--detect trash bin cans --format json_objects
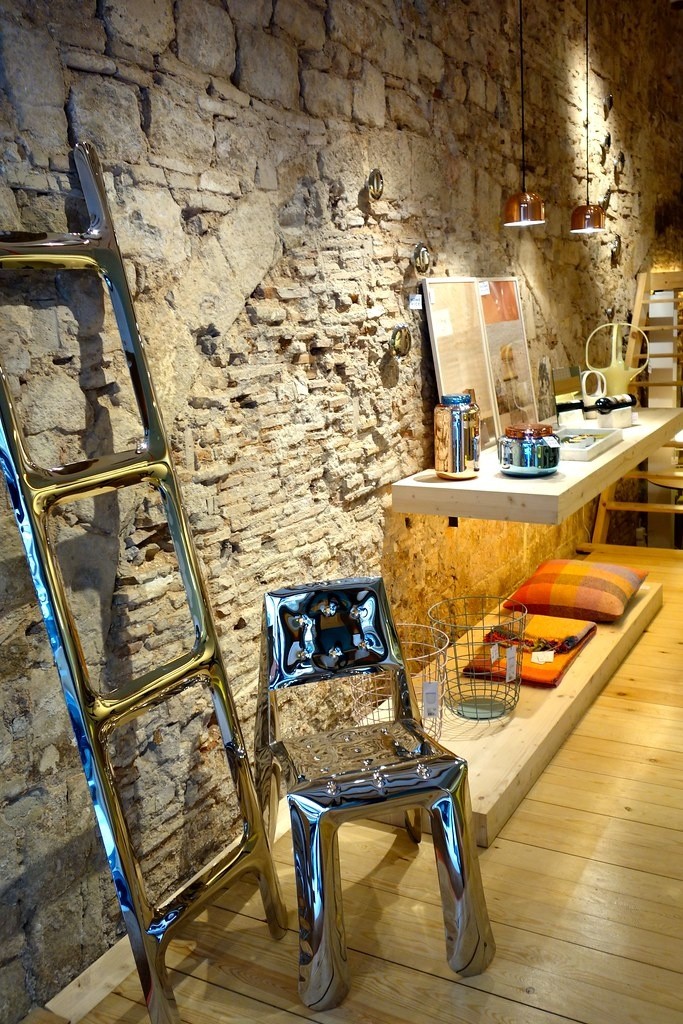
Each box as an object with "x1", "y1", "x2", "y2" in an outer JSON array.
[
  {"x1": 352, "y1": 625, "x2": 450, "y2": 745},
  {"x1": 426, "y1": 596, "x2": 528, "y2": 721}
]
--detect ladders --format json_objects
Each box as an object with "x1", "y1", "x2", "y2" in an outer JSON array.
[
  {"x1": 592, "y1": 272, "x2": 683, "y2": 558},
  {"x1": 0, "y1": 138, "x2": 292, "y2": 1024}
]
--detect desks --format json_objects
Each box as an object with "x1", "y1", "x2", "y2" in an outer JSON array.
[{"x1": 388, "y1": 405, "x2": 683, "y2": 524}]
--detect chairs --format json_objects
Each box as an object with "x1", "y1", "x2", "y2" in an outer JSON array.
[{"x1": 242, "y1": 576, "x2": 494, "y2": 1011}]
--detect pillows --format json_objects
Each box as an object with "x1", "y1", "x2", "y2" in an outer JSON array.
[{"x1": 506, "y1": 559, "x2": 650, "y2": 623}]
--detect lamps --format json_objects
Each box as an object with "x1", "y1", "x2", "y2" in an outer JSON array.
[
  {"x1": 503, "y1": 0, "x2": 545, "y2": 226},
  {"x1": 572, "y1": 0, "x2": 607, "y2": 233}
]
